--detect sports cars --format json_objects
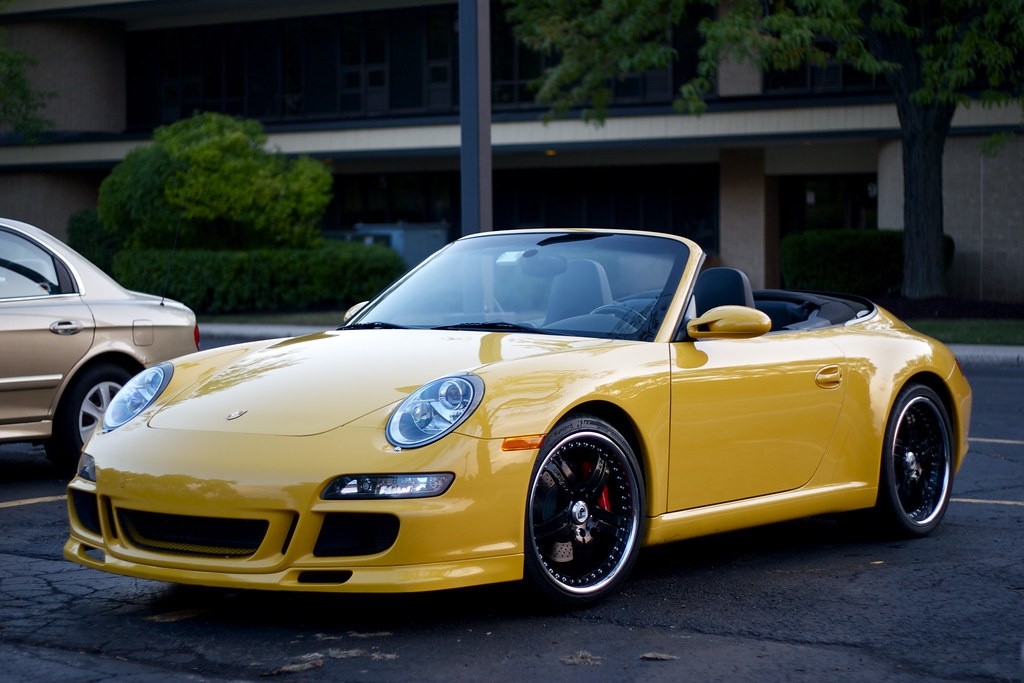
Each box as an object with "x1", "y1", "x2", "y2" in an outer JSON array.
[{"x1": 64, "y1": 225, "x2": 974, "y2": 613}]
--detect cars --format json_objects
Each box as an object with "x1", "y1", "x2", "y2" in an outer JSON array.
[{"x1": 1, "y1": 218, "x2": 201, "y2": 486}]
